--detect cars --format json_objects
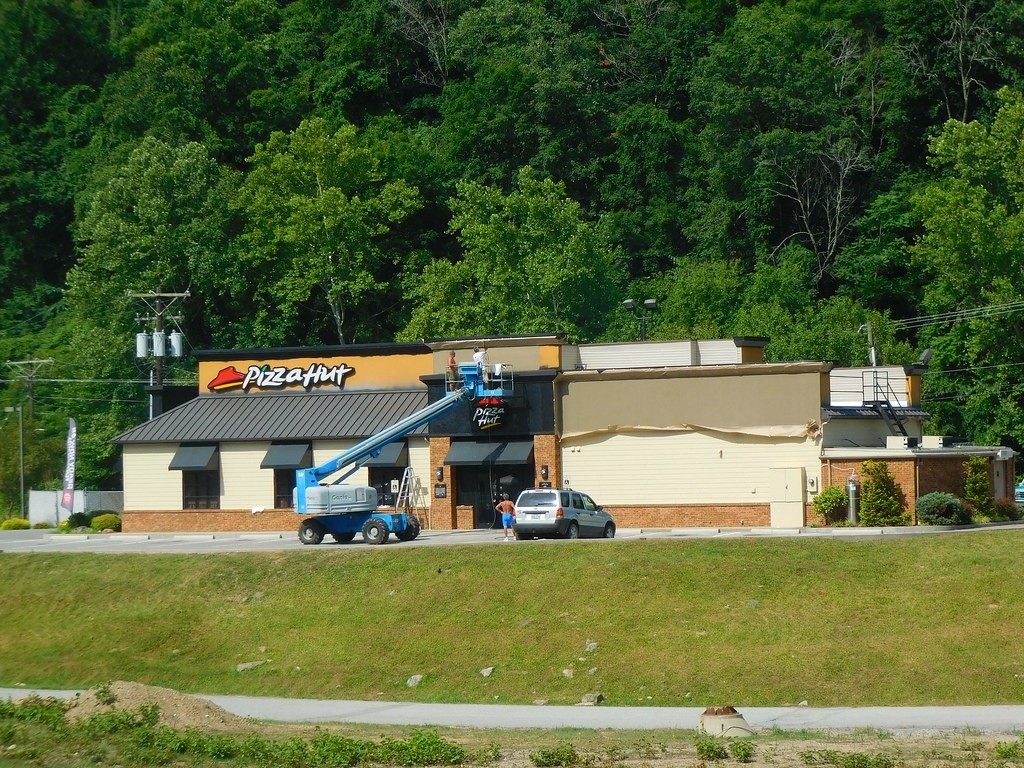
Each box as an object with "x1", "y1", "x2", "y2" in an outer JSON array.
[{"x1": 1013, "y1": 477, "x2": 1024, "y2": 505}]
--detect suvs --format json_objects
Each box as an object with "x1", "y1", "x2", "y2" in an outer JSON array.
[{"x1": 511, "y1": 488, "x2": 616, "y2": 542}]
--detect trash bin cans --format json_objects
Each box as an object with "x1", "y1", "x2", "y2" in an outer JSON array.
[{"x1": 845, "y1": 475, "x2": 860, "y2": 524}]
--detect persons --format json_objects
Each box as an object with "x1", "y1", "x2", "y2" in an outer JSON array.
[
  {"x1": 473, "y1": 346, "x2": 489, "y2": 389},
  {"x1": 447, "y1": 351, "x2": 461, "y2": 392},
  {"x1": 495, "y1": 493, "x2": 517, "y2": 541}
]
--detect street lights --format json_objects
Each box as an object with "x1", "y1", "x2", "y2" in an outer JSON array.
[{"x1": 623, "y1": 298, "x2": 659, "y2": 342}]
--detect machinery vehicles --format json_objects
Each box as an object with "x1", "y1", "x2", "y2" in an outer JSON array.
[{"x1": 293, "y1": 362, "x2": 516, "y2": 545}]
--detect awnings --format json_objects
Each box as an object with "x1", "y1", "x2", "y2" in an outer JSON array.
[
  {"x1": 354, "y1": 441, "x2": 409, "y2": 467},
  {"x1": 168, "y1": 442, "x2": 219, "y2": 470},
  {"x1": 260, "y1": 442, "x2": 312, "y2": 469},
  {"x1": 443, "y1": 440, "x2": 534, "y2": 465}
]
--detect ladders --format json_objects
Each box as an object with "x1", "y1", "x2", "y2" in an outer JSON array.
[{"x1": 394, "y1": 467, "x2": 429, "y2": 531}]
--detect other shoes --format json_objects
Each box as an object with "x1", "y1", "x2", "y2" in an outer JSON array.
[
  {"x1": 512, "y1": 536, "x2": 516, "y2": 540},
  {"x1": 504, "y1": 537, "x2": 509, "y2": 542}
]
